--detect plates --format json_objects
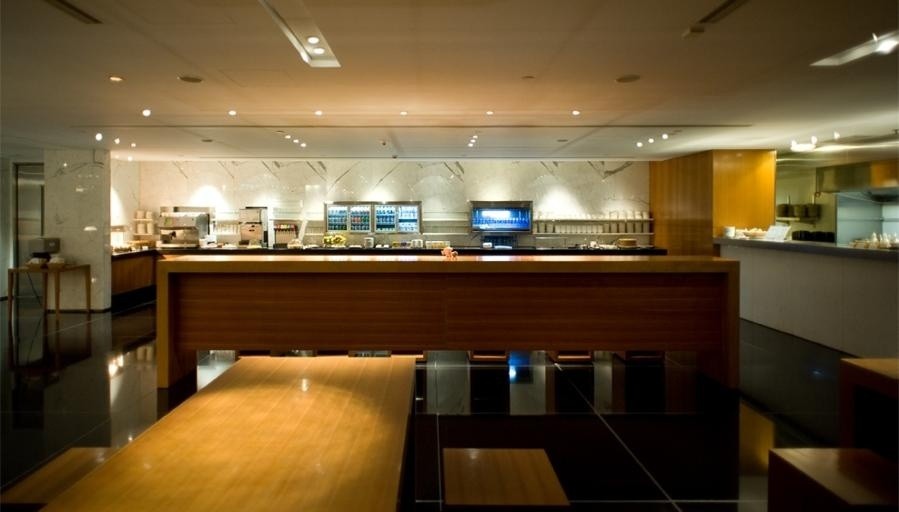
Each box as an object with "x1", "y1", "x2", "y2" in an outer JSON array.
[
  {"x1": 363, "y1": 238, "x2": 374, "y2": 249},
  {"x1": 135, "y1": 209, "x2": 154, "y2": 234},
  {"x1": 742, "y1": 231, "x2": 767, "y2": 239},
  {"x1": 410, "y1": 239, "x2": 423, "y2": 248}
]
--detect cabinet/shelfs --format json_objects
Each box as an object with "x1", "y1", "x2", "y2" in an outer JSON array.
[{"x1": 324, "y1": 201, "x2": 424, "y2": 236}]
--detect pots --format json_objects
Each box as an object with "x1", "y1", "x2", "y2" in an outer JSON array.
[{"x1": 776, "y1": 203, "x2": 822, "y2": 218}]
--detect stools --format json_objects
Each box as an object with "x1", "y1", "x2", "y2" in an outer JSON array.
[
  {"x1": 0, "y1": 443, "x2": 123, "y2": 509},
  {"x1": 770, "y1": 447, "x2": 898, "y2": 512},
  {"x1": 441, "y1": 447, "x2": 571, "y2": 509}
]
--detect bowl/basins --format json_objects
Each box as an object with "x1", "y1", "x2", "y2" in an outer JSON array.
[{"x1": 722, "y1": 225, "x2": 735, "y2": 239}]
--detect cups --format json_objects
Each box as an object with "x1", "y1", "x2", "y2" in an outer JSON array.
[
  {"x1": 534, "y1": 209, "x2": 649, "y2": 233},
  {"x1": 426, "y1": 240, "x2": 451, "y2": 250}
]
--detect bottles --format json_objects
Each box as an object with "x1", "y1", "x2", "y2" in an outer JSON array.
[
  {"x1": 473, "y1": 209, "x2": 530, "y2": 231},
  {"x1": 327, "y1": 205, "x2": 418, "y2": 230},
  {"x1": 870, "y1": 230, "x2": 899, "y2": 249}
]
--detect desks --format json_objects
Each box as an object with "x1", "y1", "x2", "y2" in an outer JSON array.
[
  {"x1": 840, "y1": 358, "x2": 899, "y2": 447},
  {"x1": 7, "y1": 264, "x2": 90, "y2": 321},
  {"x1": 36, "y1": 355, "x2": 417, "y2": 512}
]
